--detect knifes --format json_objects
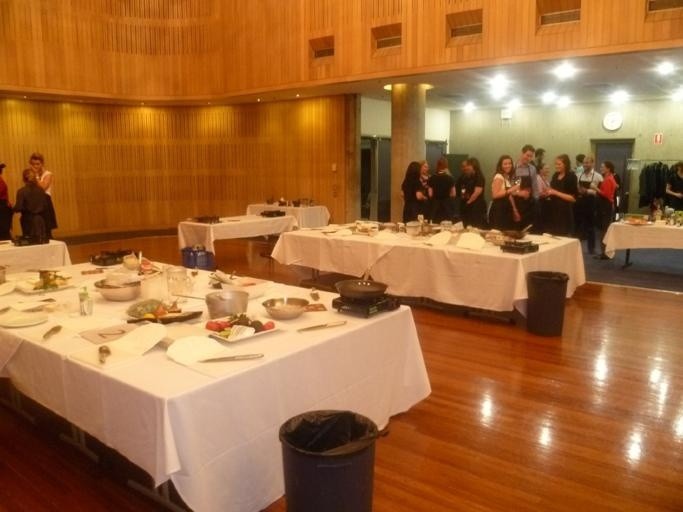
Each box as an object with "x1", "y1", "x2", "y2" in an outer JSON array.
[
  {"x1": 297, "y1": 320, "x2": 347, "y2": 331},
  {"x1": 199, "y1": 353, "x2": 264, "y2": 362}
]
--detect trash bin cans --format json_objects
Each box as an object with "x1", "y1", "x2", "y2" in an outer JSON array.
[
  {"x1": 278, "y1": 409, "x2": 377, "y2": 512},
  {"x1": 527, "y1": 271, "x2": 569, "y2": 337}
]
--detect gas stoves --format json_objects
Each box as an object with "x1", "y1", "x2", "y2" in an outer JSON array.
[
  {"x1": 91, "y1": 250, "x2": 138, "y2": 266},
  {"x1": 10, "y1": 235, "x2": 53, "y2": 244},
  {"x1": 260, "y1": 209, "x2": 287, "y2": 217},
  {"x1": 333, "y1": 295, "x2": 398, "y2": 316},
  {"x1": 503, "y1": 242, "x2": 539, "y2": 253}
]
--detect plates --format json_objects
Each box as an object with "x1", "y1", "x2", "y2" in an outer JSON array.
[
  {"x1": 198, "y1": 311, "x2": 280, "y2": 343},
  {"x1": 0, "y1": 311, "x2": 49, "y2": 327},
  {"x1": 17, "y1": 282, "x2": 73, "y2": 294},
  {"x1": 142, "y1": 263, "x2": 163, "y2": 279},
  {"x1": 0, "y1": 281, "x2": 16, "y2": 295}
]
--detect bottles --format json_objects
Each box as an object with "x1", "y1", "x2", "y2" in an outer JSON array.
[{"x1": 79, "y1": 286, "x2": 93, "y2": 317}]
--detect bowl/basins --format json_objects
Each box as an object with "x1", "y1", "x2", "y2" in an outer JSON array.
[
  {"x1": 222, "y1": 279, "x2": 275, "y2": 298},
  {"x1": 94, "y1": 279, "x2": 141, "y2": 302},
  {"x1": 262, "y1": 298, "x2": 309, "y2": 320},
  {"x1": 405, "y1": 222, "x2": 423, "y2": 236},
  {"x1": 433, "y1": 227, "x2": 440, "y2": 234},
  {"x1": 503, "y1": 229, "x2": 527, "y2": 239},
  {"x1": 205, "y1": 291, "x2": 249, "y2": 319}
]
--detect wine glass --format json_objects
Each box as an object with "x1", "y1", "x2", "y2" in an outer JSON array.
[{"x1": 514, "y1": 176, "x2": 521, "y2": 192}]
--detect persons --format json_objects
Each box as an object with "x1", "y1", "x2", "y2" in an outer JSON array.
[
  {"x1": 401, "y1": 161, "x2": 431, "y2": 197},
  {"x1": 401, "y1": 162, "x2": 428, "y2": 223},
  {"x1": 575, "y1": 154, "x2": 584, "y2": 178},
  {"x1": 590, "y1": 161, "x2": 617, "y2": 260},
  {"x1": 0, "y1": 164, "x2": 13, "y2": 240},
  {"x1": 601, "y1": 172, "x2": 621, "y2": 222},
  {"x1": 489, "y1": 155, "x2": 521, "y2": 229},
  {"x1": 546, "y1": 154, "x2": 579, "y2": 237},
  {"x1": 28, "y1": 153, "x2": 58, "y2": 240},
  {"x1": 665, "y1": 161, "x2": 683, "y2": 211},
  {"x1": 466, "y1": 157, "x2": 488, "y2": 225},
  {"x1": 427, "y1": 157, "x2": 456, "y2": 223},
  {"x1": 455, "y1": 159, "x2": 469, "y2": 225},
  {"x1": 576, "y1": 157, "x2": 604, "y2": 253},
  {"x1": 513, "y1": 144, "x2": 546, "y2": 230},
  {"x1": 536, "y1": 163, "x2": 551, "y2": 193},
  {"x1": 535, "y1": 149, "x2": 545, "y2": 166},
  {"x1": 12, "y1": 169, "x2": 49, "y2": 240}
]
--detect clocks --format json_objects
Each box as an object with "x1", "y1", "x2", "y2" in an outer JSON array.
[{"x1": 603, "y1": 112, "x2": 623, "y2": 131}]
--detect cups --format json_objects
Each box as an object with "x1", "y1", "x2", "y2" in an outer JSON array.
[
  {"x1": 167, "y1": 266, "x2": 192, "y2": 303},
  {"x1": 439, "y1": 220, "x2": 452, "y2": 232}
]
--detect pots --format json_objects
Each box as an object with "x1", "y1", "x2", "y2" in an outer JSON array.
[{"x1": 334, "y1": 270, "x2": 388, "y2": 299}]
